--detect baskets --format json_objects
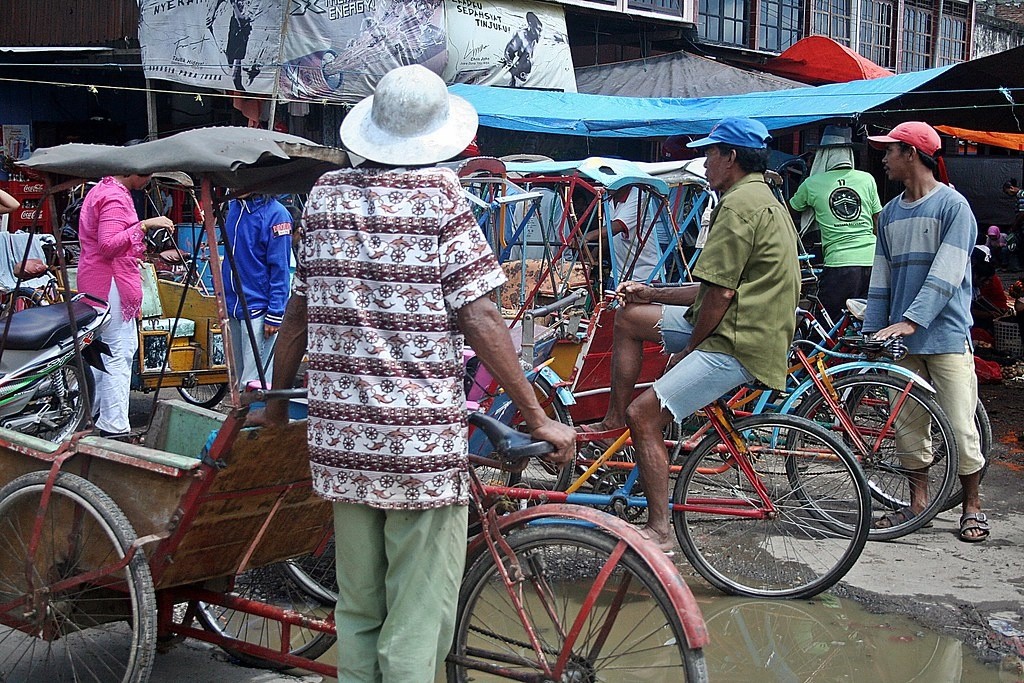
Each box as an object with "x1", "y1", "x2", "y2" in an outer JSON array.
[{"x1": 994, "y1": 315, "x2": 1023, "y2": 355}]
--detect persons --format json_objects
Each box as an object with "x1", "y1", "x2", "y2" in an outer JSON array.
[
  {"x1": 206, "y1": 0, "x2": 261, "y2": 80},
  {"x1": 862, "y1": 121, "x2": 990, "y2": 541},
  {"x1": 785, "y1": 125, "x2": 882, "y2": 345},
  {"x1": 263, "y1": 64, "x2": 575, "y2": 683},
  {"x1": 222, "y1": 185, "x2": 294, "y2": 396},
  {"x1": 484, "y1": 134, "x2": 690, "y2": 292},
  {"x1": 161, "y1": 187, "x2": 173, "y2": 218},
  {"x1": 504, "y1": 11, "x2": 543, "y2": 87},
  {"x1": 971, "y1": 245, "x2": 1008, "y2": 322},
  {"x1": 985, "y1": 181, "x2": 1024, "y2": 273},
  {"x1": 77, "y1": 172, "x2": 175, "y2": 443},
  {"x1": 574, "y1": 115, "x2": 801, "y2": 558},
  {"x1": 0, "y1": 189, "x2": 20, "y2": 214}
]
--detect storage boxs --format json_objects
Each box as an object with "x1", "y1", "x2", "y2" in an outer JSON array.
[{"x1": 0, "y1": 179, "x2": 54, "y2": 235}]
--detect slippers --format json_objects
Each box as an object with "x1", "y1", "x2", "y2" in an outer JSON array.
[
  {"x1": 875, "y1": 507, "x2": 934, "y2": 529},
  {"x1": 960, "y1": 511, "x2": 991, "y2": 542},
  {"x1": 581, "y1": 425, "x2": 625, "y2": 457}
]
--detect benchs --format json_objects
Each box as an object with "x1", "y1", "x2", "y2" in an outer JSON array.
[
  {"x1": 551, "y1": 300, "x2": 674, "y2": 419},
  {"x1": 56, "y1": 261, "x2": 199, "y2": 336},
  {"x1": 63, "y1": 382, "x2": 343, "y2": 592}
]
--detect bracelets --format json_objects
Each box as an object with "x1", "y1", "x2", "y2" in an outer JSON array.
[{"x1": 140, "y1": 222, "x2": 147, "y2": 233}]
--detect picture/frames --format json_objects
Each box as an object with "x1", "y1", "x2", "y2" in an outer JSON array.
[
  {"x1": 137, "y1": 329, "x2": 172, "y2": 373},
  {"x1": 208, "y1": 328, "x2": 228, "y2": 369}
]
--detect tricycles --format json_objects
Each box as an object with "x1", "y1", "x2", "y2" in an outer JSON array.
[{"x1": 0, "y1": 125, "x2": 993, "y2": 683}]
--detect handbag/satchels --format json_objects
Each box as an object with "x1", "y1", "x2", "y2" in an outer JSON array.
[{"x1": 136, "y1": 261, "x2": 163, "y2": 318}]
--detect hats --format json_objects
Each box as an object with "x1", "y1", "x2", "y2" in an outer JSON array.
[
  {"x1": 685, "y1": 117, "x2": 771, "y2": 150},
  {"x1": 866, "y1": 121, "x2": 943, "y2": 164},
  {"x1": 340, "y1": 64, "x2": 478, "y2": 166},
  {"x1": 807, "y1": 126, "x2": 864, "y2": 147}
]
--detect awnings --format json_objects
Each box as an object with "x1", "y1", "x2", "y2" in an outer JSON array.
[{"x1": 749, "y1": 35, "x2": 898, "y2": 85}]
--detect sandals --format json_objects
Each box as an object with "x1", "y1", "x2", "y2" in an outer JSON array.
[{"x1": 100, "y1": 429, "x2": 146, "y2": 444}]
